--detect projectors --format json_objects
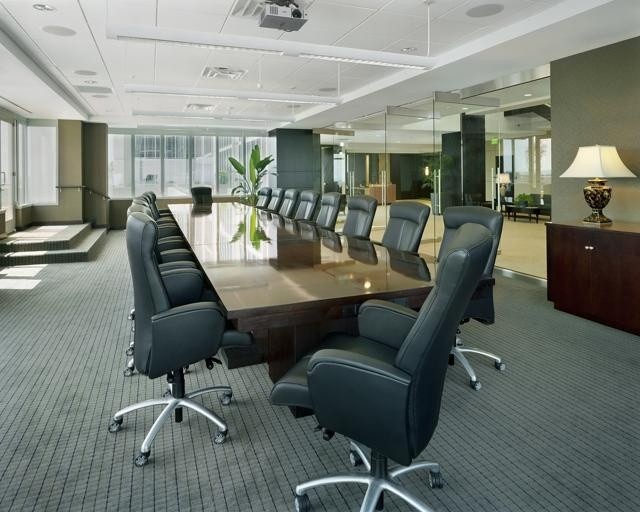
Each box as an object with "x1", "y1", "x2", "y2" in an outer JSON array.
[{"x1": 258, "y1": 5, "x2": 311, "y2": 33}]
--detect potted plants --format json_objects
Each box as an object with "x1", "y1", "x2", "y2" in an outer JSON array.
[{"x1": 514, "y1": 193, "x2": 536, "y2": 208}]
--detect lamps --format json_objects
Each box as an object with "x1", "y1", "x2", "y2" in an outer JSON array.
[{"x1": 559, "y1": 143, "x2": 637, "y2": 227}]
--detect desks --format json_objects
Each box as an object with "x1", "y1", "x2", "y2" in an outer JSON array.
[{"x1": 167, "y1": 201, "x2": 495, "y2": 420}]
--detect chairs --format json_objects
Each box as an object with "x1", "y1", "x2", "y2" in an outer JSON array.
[
  {"x1": 316, "y1": 191, "x2": 342, "y2": 228},
  {"x1": 191, "y1": 188, "x2": 213, "y2": 203},
  {"x1": 266, "y1": 223, "x2": 494, "y2": 511},
  {"x1": 381, "y1": 199, "x2": 431, "y2": 252},
  {"x1": 437, "y1": 205, "x2": 505, "y2": 391},
  {"x1": 108, "y1": 191, "x2": 234, "y2": 467},
  {"x1": 342, "y1": 195, "x2": 379, "y2": 237},
  {"x1": 256, "y1": 188, "x2": 320, "y2": 221}
]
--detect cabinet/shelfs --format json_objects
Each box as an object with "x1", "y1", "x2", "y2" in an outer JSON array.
[{"x1": 543, "y1": 220, "x2": 640, "y2": 336}]
